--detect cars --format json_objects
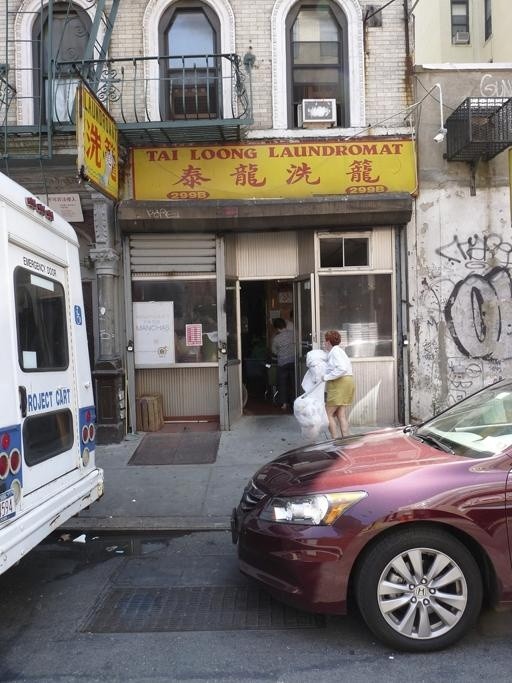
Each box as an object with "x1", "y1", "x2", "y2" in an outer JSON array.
[{"x1": 228, "y1": 375, "x2": 510, "y2": 658}]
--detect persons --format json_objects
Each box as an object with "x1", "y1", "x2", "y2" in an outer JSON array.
[
  {"x1": 320, "y1": 330, "x2": 355, "y2": 439},
  {"x1": 270, "y1": 318, "x2": 298, "y2": 409},
  {"x1": 282, "y1": 308, "x2": 294, "y2": 330}
]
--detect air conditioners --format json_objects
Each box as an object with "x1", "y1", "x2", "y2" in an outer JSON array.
[
  {"x1": 454, "y1": 31, "x2": 470, "y2": 43},
  {"x1": 298, "y1": 98, "x2": 338, "y2": 128}
]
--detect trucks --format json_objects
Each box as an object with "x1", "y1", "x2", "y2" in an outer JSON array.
[{"x1": 1, "y1": 172, "x2": 106, "y2": 576}]
